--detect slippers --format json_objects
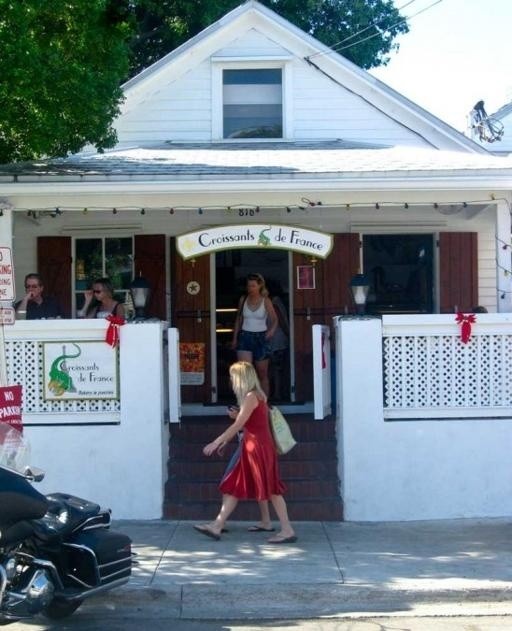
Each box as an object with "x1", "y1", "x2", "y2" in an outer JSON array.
[
  {"x1": 247, "y1": 525, "x2": 277, "y2": 534},
  {"x1": 190, "y1": 525, "x2": 221, "y2": 543},
  {"x1": 265, "y1": 534, "x2": 299, "y2": 546}
]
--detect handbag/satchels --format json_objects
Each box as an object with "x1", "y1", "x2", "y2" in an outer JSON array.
[{"x1": 267, "y1": 402, "x2": 297, "y2": 457}]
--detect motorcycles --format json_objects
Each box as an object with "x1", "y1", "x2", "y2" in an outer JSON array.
[{"x1": 0, "y1": 420, "x2": 139, "y2": 625}]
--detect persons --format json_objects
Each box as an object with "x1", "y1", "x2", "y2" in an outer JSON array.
[
  {"x1": 230, "y1": 272, "x2": 279, "y2": 403},
  {"x1": 217, "y1": 399, "x2": 276, "y2": 532},
  {"x1": 190, "y1": 360, "x2": 297, "y2": 545},
  {"x1": 11, "y1": 273, "x2": 60, "y2": 320},
  {"x1": 261, "y1": 294, "x2": 290, "y2": 402},
  {"x1": 76, "y1": 277, "x2": 125, "y2": 319}
]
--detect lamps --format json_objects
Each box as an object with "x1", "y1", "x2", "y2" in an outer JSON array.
[
  {"x1": 130, "y1": 270, "x2": 153, "y2": 321},
  {"x1": 347, "y1": 269, "x2": 372, "y2": 316}
]
